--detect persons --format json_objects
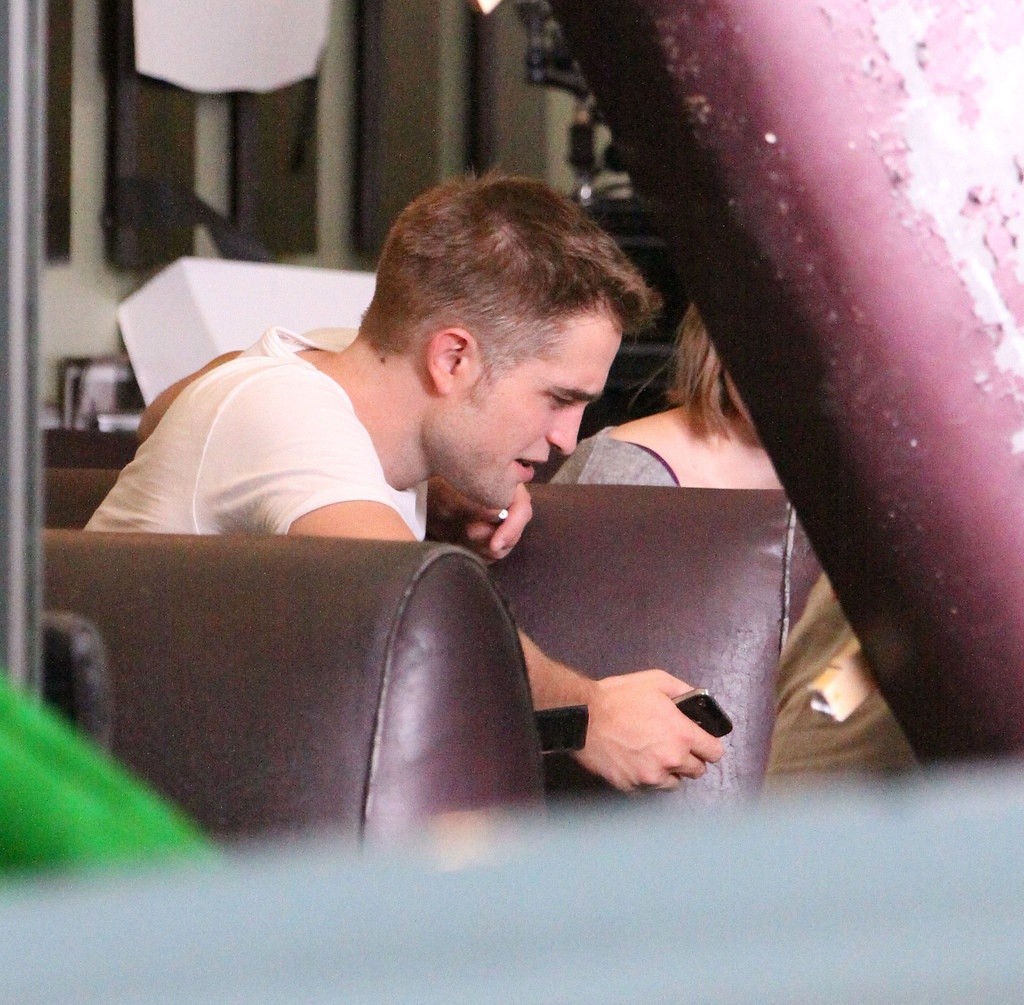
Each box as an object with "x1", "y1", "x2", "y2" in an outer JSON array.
[
  {"x1": 78, "y1": 177, "x2": 725, "y2": 796},
  {"x1": 546, "y1": 301, "x2": 786, "y2": 489}
]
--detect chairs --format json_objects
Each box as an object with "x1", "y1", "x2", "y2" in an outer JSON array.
[
  {"x1": 41, "y1": 528, "x2": 546, "y2": 879},
  {"x1": 421, "y1": 482, "x2": 825, "y2": 812}
]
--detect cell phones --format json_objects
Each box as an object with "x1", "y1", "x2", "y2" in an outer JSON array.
[{"x1": 671, "y1": 689, "x2": 733, "y2": 737}]
